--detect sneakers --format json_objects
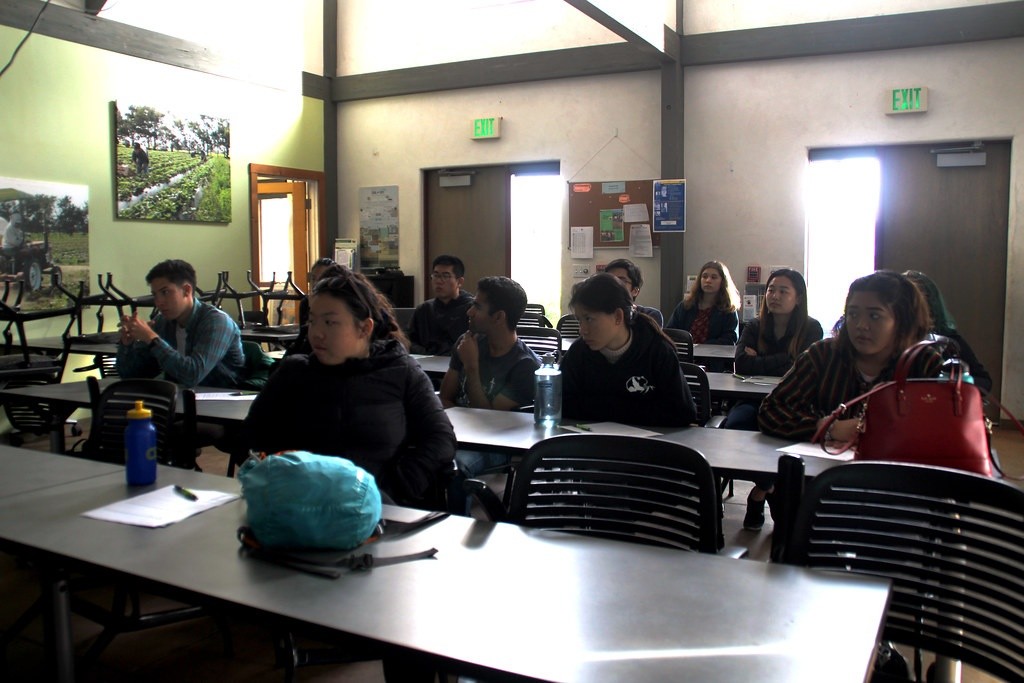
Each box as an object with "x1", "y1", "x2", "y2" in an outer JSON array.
[{"x1": 742, "y1": 491, "x2": 766, "y2": 531}]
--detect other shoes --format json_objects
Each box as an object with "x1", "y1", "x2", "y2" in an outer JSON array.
[{"x1": 871, "y1": 651, "x2": 913, "y2": 683}]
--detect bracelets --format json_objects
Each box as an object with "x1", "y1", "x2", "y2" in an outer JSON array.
[{"x1": 149, "y1": 336, "x2": 162, "y2": 348}]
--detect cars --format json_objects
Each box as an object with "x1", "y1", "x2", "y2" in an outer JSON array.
[{"x1": 0, "y1": 187, "x2": 63, "y2": 297}]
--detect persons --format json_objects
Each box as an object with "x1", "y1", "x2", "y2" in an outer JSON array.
[
  {"x1": 201, "y1": 151, "x2": 206, "y2": 161},
  {"x1": 132, "y1": 143, "x2": 149, "y2": 175},
  {"x1": 411, "y1": 256, "x2": 479, "y2": 355},
  {"x1": 757, "y1": 270, "x2": 992, "y2": 442},
  {"x1": 561, "y1": 258, "x2": 697, "y2": 428},
  {"x1": 725, "y1": 269, "x2": 823, "y2": 531},
  {"x1": 228, "y1": 256, "x2": 456, "y2": 516},
  {"x1": 670, "y1": 262, "x2": 737, "y2": 390},
  {"x1": 116, "y1": 260, "x2": 246, "y2": 471},
  {"x1": 439, "y1": 276, "x2": 540, "y2": 516},
  {"x1": 3, "y1": 213, "x2": 31, "y2": 249}
]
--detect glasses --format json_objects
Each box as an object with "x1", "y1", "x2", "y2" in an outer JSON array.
[{"x1": 430, "y1": 272, "x2": 458, "y2": 282}]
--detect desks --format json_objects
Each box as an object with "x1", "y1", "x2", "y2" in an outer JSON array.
[{"x1": 0, "y1": 327, "x2": 894, "y2": 683}]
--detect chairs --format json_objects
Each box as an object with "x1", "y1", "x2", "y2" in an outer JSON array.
[
  {"x1": 0, "y1": 267, "x2": 966, "y2": 560},
  {"x1": 768, "y1": 451, "x2": 1024, "y2": 683}
]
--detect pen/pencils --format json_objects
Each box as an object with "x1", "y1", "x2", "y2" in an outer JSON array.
[
  {"x1": 733, "y1": 373, "x2": 745, "y2": 380},
  {"x1": 569, "y1": 421, "x2": 591, "y2": 432},
  {"x1": 175, "y1": 485, "x2": 197, "y2": 500},
  {"x1": 230, "y1": 391, "x2": 258, "y2": 396}
]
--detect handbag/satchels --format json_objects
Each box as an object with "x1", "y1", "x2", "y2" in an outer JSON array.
[
  {"x1": 812, "y1": 341, "x2": 1024, "y2": 481},
  {"x1": 240, "y1": 449, "x2": 381, "y2": 550}
]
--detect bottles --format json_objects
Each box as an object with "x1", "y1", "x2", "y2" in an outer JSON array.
[
  {"x1": 126, "y1": 401, "x2": 156, "y2": 485},
  {"x1": 535, "y1": 353, "x2": 562, "y2": 426},
  {"x1": 939, "y1": 358, "x2": 974, "y2": 385}
]
